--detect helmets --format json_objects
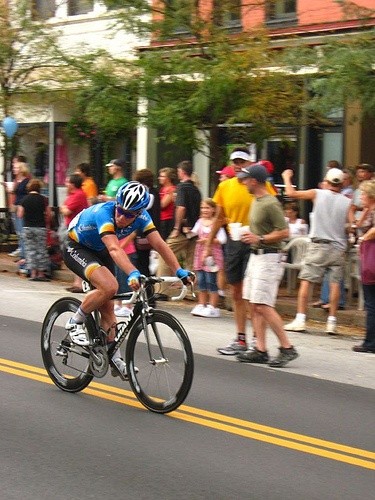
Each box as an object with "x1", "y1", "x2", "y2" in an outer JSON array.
[{"x1": 116, "y1": 181, "x2": 150, "y2": 212}]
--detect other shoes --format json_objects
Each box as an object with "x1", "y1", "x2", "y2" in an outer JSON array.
[
  {"x1": 191, "y1": 304, "x2": 204, "y2": 316},
  {"x1": 8, "y1": 247, "x2": 22, "y2": 258},
  {"x1": 65, "y1": 286, "x2": 79, "y2": 292},
  {"x1": 66, "y1": 318, "x2": 90, "y2": 345},
  {"x1": 28, "y1": 277, "x2": 36, "y2": 280},
  {"x1": 284, "y1": 318, "x2": 307, "y2": 332},
  {"x1": 110, "y1": 357, "x2": 140, "y2": 379},
  {"x1": 235, "y1": 347, "x2": 270, "y2": 364},
  {"x1": 352, "y1": 344, "x2": 375, "y2": 353},
  {"x1": 269, "y1": 345, "x2": 299, "y2": 368},
  {"x1": 217, "y1": 339, "x2": 247, "y2": 356},
  {"x1": 199, "y1": 305, "x2": 220, "y2": 318},
  {"x1": 70, "y1": 288, "x2": 86, "y2": 293},
  {"x1": 36, "y1": 277, "x2": 50, "y2": 282},
  {"x1": 326, "y1": 322, "x2": 337, "y2": 334}
]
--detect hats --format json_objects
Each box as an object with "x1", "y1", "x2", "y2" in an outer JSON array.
[
  {"x1": 326, "y1": 168, "x2": 344, "y2": 184},
  {"x1": 238, "y1": 164, "x2": 267, "y2": 181},
  {"x1": 257, "y1": 161, "x2": 273, "y2": 175},
  {"x1": 215, "y1": 165, "x2": 236, "y2": 176},
  {"x1": 229, "y1": 151, "x2": 250, "y2": 161},
  {"x1": 356, "y1": 163, "x2": 372, "y2": 170}
]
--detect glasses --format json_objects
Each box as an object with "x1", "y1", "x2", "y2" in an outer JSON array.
[{"x1": 116, "y1": 205, "x2": 137, "y2": 219}]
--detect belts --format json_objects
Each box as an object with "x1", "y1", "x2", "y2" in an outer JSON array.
[{"x1": 250, "y1": 248, "x2": 281, "y2": 255}]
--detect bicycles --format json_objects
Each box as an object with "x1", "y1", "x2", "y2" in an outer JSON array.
[{"x1": 41, "y1": 272, "x2": 197, "y2": 414}]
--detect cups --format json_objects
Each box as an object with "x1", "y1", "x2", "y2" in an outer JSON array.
[
  {"x1": 5, "y1": 182, "x2": 15, "y2": 191},
  {"x1": 238, "y1": 225, "x2": 250, "y2": 237},
  {"x1": 228, "y1": 223, "x2": 242, "y2": 241}
]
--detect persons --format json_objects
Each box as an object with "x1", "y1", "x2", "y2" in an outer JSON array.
[
  {"x1": 202, "y1": 146, "x2": 276, "y2": 355},
  {"x1": 62, "y1": 180, "x2": 195, "y2": 376},
  {"x1": 61, "y1": 159, "x2": 375, "y2": 353},
  {"x1": 236, "y1": 164, "x2": 299, "y2": 367},
  {"x1": 0, "y1": 156, "x2": 63, "y2": 281}
]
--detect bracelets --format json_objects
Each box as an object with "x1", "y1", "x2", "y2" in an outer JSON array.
[
  {"x1": 173, "y1": 226, "x2": 180, "y2": 230},
  {"x1": 357, "y1": 236, "x2": 365, "y2": 242}
]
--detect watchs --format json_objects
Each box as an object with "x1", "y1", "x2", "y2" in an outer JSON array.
[{"x1": 259, "y1": 235, "x2": 264, "y2": 244}]
